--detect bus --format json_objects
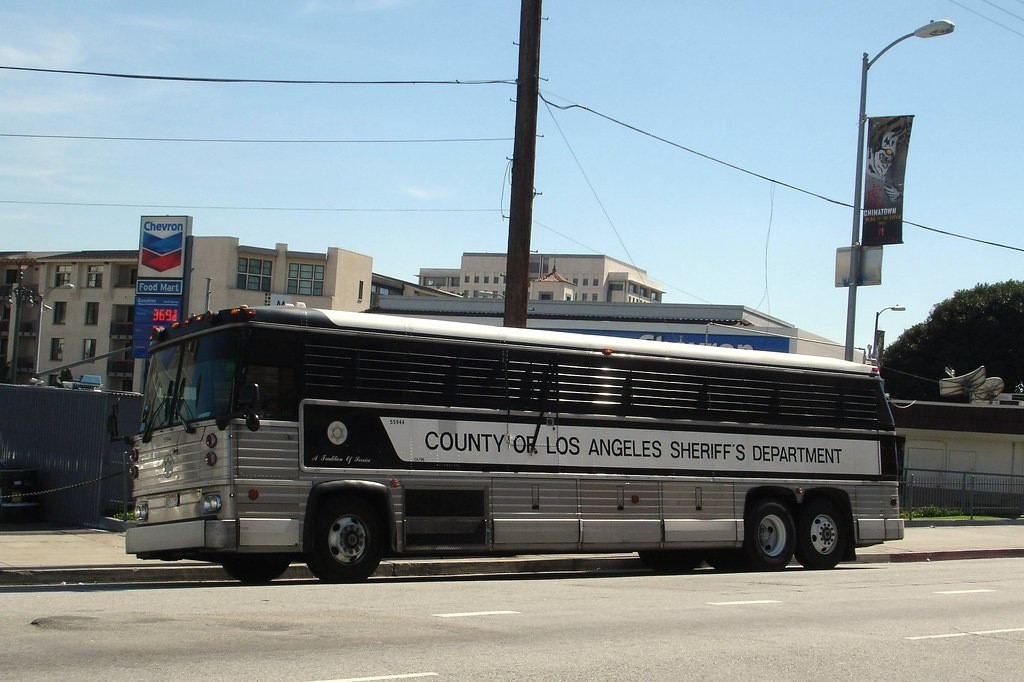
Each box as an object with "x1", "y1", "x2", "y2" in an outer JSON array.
[{"x1": 107, "y1": 303, "x2": 904, "y2": 582}]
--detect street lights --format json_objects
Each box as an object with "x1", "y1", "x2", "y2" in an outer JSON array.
[
  {"x1": 844, "y1": 16, "x2": 956, "y2": 358},
  {"x1": 872, "y1": 306, "x2": 906, "y2": 358},
  {"x1": 35, "y1": 284, "x2": 74, "y2": 382},
  {"x1": 5, "y1": 287, "x2": 35, "y2": 384}
]
